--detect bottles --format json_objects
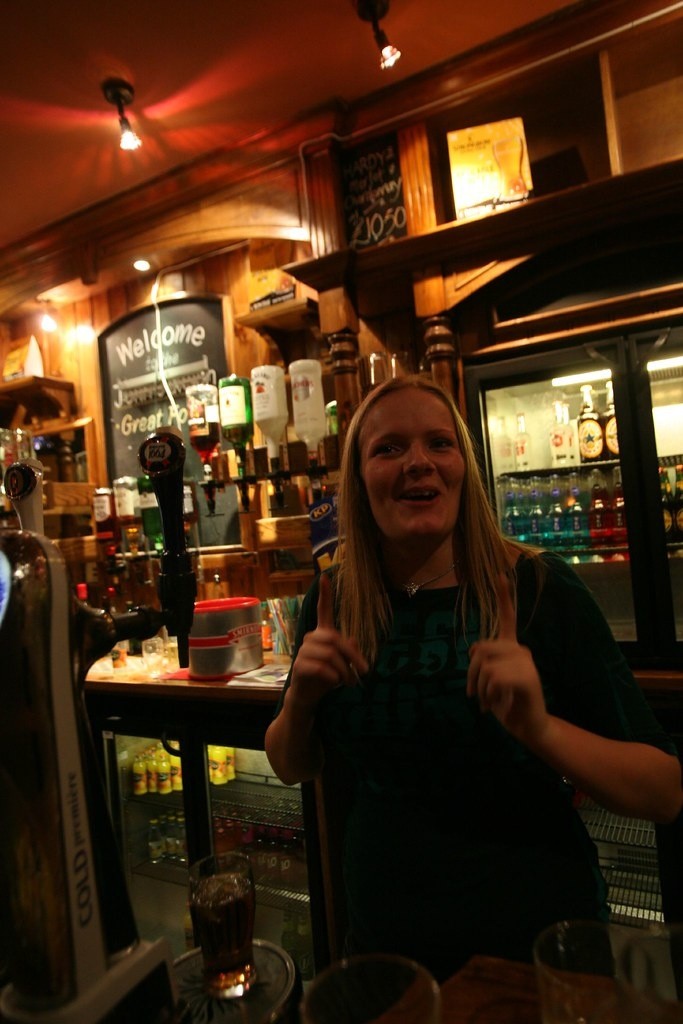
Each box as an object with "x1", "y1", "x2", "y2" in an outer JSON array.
[
  {"x1": 138, "y1": 476, "x2": 165, "y2": 561},
  {"x1": 251, "y1": 366, "x2": 288, "y2": 473},
  {"x1": 261, "y1": 602, "x2": 273, "y2": 650},
  {"x1": 219, "y1": 378, "x2": 254, "y2": 476},
  {"x1": 132, "y1": 741, "x2": 304, "y2": 884},
  {"x1": 550, "y1": 401, "x2": 575, "y2": 465},
  {"x1": 659, "y1": 465, "x2": 683, "y2": 558},
  {"x1": 290, "y1": 359, "x2": 327, "y2": 470},
  {"x1": 578, "y1": 385, "x2": 609, "y2": 462},
  {"x1": 503, "y1": 467, "x2": 628, "y2": 548},
  {"x1": 92, "y1": 487, "x2": 122, "y2": 560},
  {"x1": 113, "y1": 475, "x2": 142, "y2": 559},
  {"x1": 514, "y1": 411, "x2": 533, "y2": 471},
  {"x1": 186, "y1": 384, "x2": 219, "y2": 481},
  {"x1": 182, "y1": 477, "x2": 199, "y2": 546},
  {"x1": 602, "y1": 381, "x2": 621, "y2": 459}
]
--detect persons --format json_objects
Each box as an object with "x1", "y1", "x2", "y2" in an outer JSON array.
[{"x1": 263, "y1": 375, "x2": 683, "y2": 992}]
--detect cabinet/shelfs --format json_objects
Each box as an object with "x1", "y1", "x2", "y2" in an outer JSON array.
[
  {"x1": 282, "y1": 0, "x2": 683, "y2": 670},
  {"x1": 85, "y1": 657, "x2": 336, "y2": 988}
]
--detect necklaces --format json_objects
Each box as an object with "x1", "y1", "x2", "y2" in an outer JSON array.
[{"x1": 392, "y1": 553, "x2": 467, "y2": 597}]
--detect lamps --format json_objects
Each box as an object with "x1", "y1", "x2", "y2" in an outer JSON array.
[
  {"x1": 357, "y1": 0, "x2": 402, "y2": 69},
  {"x1": 102, "y1": 78, "x2": 144, "y2": 151}
]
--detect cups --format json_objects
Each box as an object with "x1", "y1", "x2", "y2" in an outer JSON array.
[
  {"x1": 369, "y1": 352, "x2": 386, "y2": 385},
  {"x1": 142, "y1": 638, "x2": 180, "y2": 678},
  {"x1": 188, "y1": 852, "x2": 257, "y2": 999},
  {"x1": 304, "y1": 954, "x2": 442, "y2": 1024},
  {"x1": 392, "y1": 355, "x2": 404, "y2": 377},
  {"x1": 1, "y1": 428, "x2": 32, "y2": 494},
  {"x1": 270, "y1": 620, "x2": 296, "y2": 656},
  {"x1": 534, "y1": 920, "x2": 648, "y2": 1023}
]
